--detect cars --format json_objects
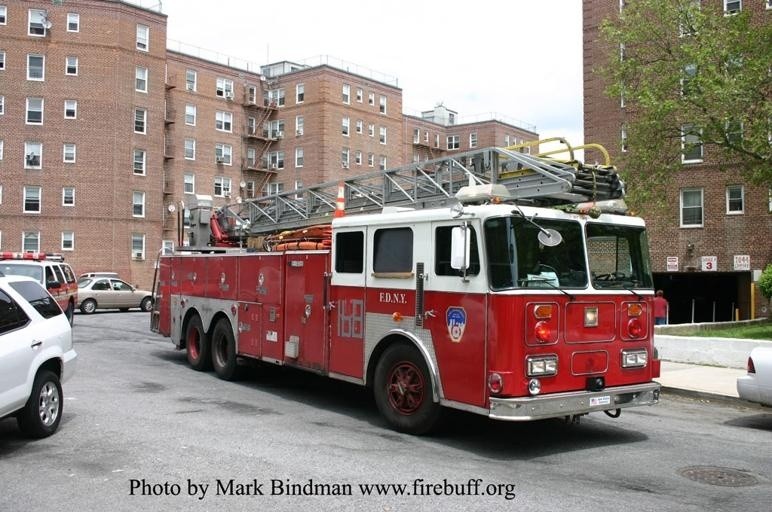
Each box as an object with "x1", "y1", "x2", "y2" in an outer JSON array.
[
  {"x1": 0, "y1": 271, "x2": 83, "y2": 440},
  {"x1": 74, "y1": 277, "x2": 159, "y2": 315}
]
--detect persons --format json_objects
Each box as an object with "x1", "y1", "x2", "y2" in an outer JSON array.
[{"x1": 654, "y1": 289, "x2": 670, "y2": 325}]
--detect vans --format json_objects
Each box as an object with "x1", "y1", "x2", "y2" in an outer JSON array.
[
  {"x1": 0, "y1": 248, "x2": 79, "y2": 328},
  {"x1": 78, "y1": 270, "x2": 122, "y2": 289}
]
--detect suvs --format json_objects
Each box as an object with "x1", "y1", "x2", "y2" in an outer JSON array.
[{"x1": 147, "y1": 137, "x2": 662, "y2": 435}]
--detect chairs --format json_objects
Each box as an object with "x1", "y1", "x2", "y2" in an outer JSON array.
[{"x1": 543, "y1": 241, "x2": 568, "y2": 269}]
